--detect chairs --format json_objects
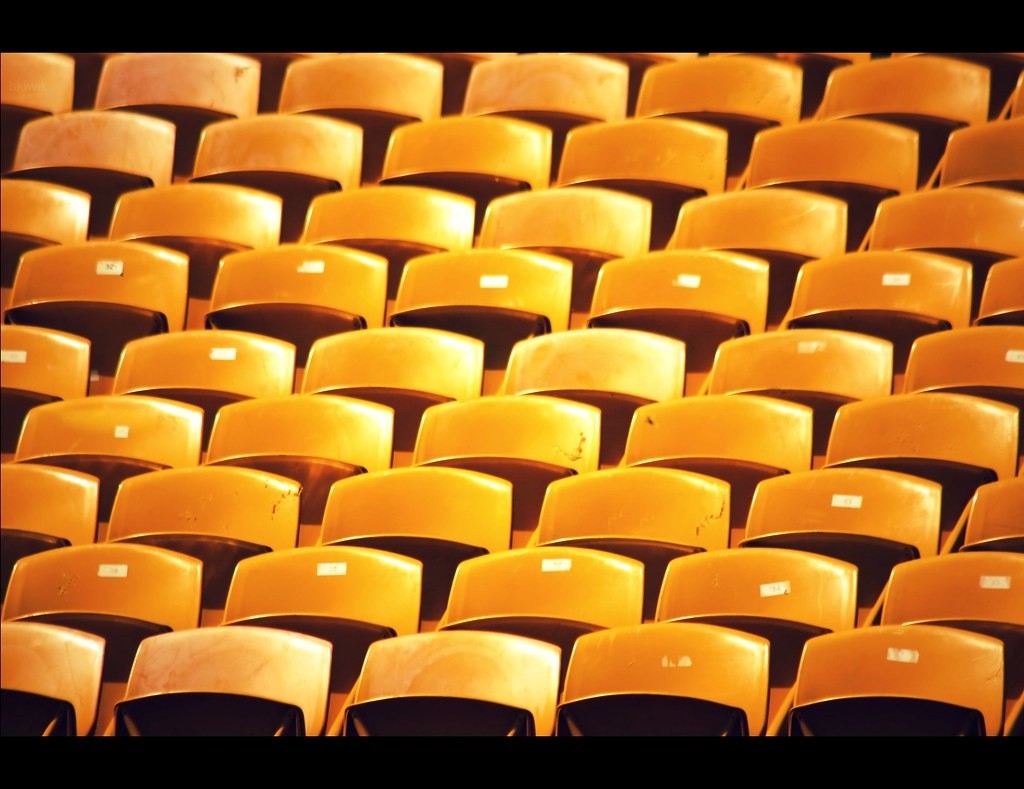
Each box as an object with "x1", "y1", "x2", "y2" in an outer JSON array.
[{"x1": 0, "y1": 53, "x2": 1024, "y2": 736}]
